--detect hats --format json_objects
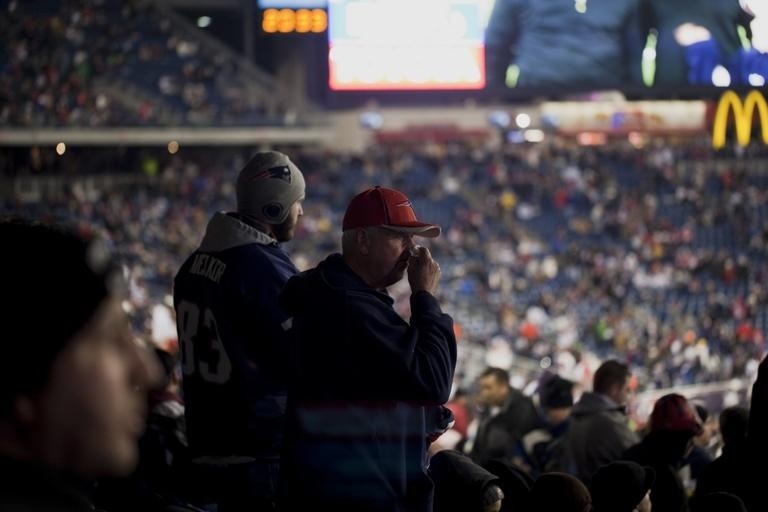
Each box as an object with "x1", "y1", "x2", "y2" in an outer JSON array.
[
  {"x1": 342, "y1": 185, "x2": 441, "y2": 240},
  {"x1": 645, "y1": 393, "x2": 705, "y2": 439}
]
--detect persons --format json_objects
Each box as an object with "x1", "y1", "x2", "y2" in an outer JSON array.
[
  {"x1": 429, "y1": 362, "x2": 768, "y2": 512},
  {"x1": 282, "y1": 184, "x2": 457, "y2": 512},
  {"x1": 0, "y1": 212, "x2": 159, "y2": 512},
  {"x1": 137, "y1": 345, "x2": 192, "y2": 512},
  {"x1": 173, "y1": 152, "x2": 306, "y2": 512},
  {"x1": 638, "y1": 1, "x2": 768, "y2": 87},
  {"x1": 485, "y1": 1, "x2": 644, "y2": 90},
  {"x1": 0, "y1": 1, "x2": 768, "y2": 427}
]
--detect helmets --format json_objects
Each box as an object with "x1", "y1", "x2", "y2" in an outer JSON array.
[{"x1": 236, "y1": 149, "x2": 306, "y2": 225}]
